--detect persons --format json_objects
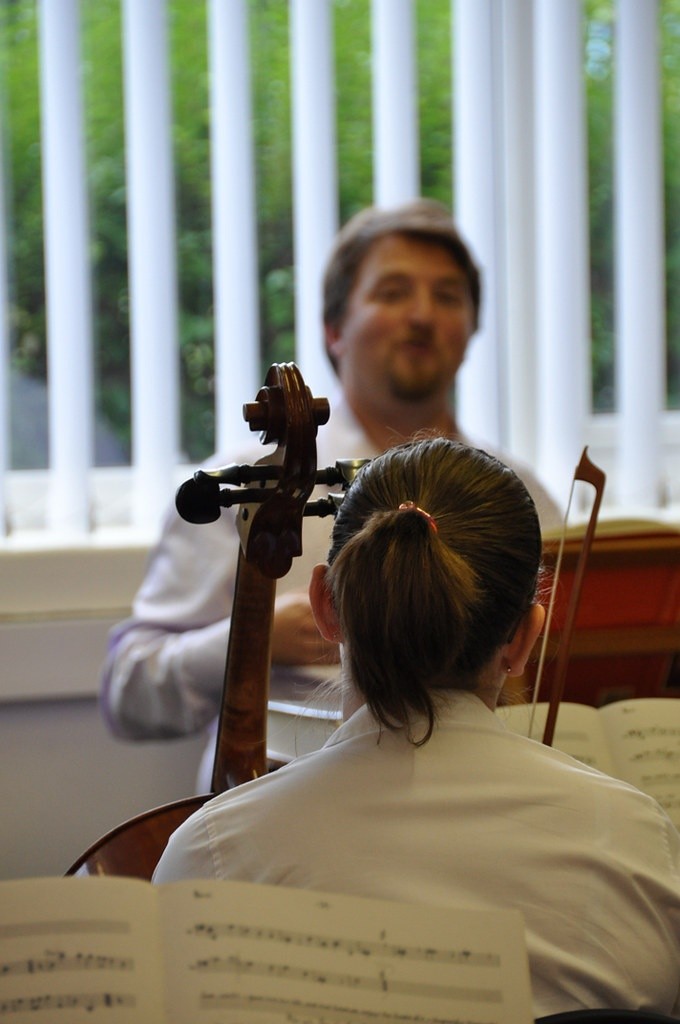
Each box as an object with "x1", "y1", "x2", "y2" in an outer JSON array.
[
  {"x1": 150, "y1": 438, "x2": 680, "y2": 1024},
  {"x1": 93, "y1": 200, "x2": 566, "y2": 797}
]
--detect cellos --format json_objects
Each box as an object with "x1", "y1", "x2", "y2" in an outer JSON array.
[{"x1": 61, "y1": 362, "x2": 373, "y2": 881}]
